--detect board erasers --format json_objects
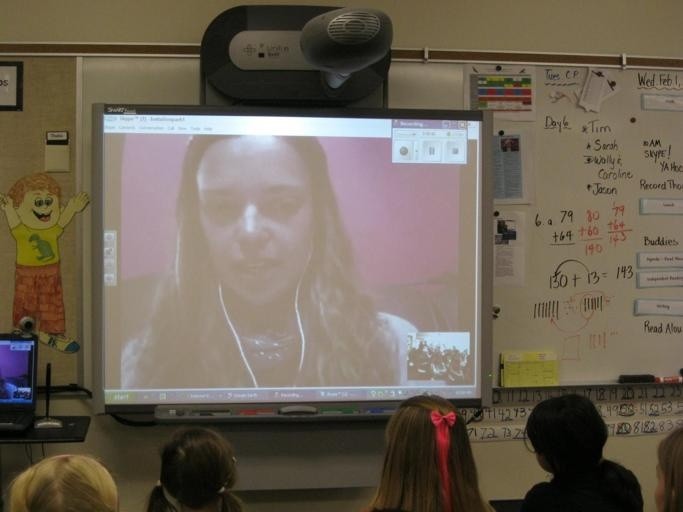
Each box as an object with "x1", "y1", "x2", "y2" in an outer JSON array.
[
  {"x1": 619, "y1": 375, "x2": 655, "y2": 383},
  {"x1": 279, "y1": 405, "x2": 317, "y2": 415}
]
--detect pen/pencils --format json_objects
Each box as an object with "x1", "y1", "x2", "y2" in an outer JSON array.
[
  {"x1": 320, "y1": 407, "x2": 399, "y2": 414},
  {"x1": 655, "y1": 376, "x2": 683, "y2": 383},
  {"x1": 169, "y1": 407, "x2": 276, "y2": 415}
]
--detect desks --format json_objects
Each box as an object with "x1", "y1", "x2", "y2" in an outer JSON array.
[{"x1": 0, "y1": 417, "x2": 91, "y2": 465}]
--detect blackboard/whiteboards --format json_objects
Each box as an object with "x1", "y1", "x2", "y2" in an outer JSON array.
[{"x1": 76, "y1": 42, "x2": 683, "y2": 394}]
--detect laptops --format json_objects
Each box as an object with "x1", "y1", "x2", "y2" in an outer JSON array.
[{"x1": 0, "y1": 333, "x2": 39, "y2": 431}]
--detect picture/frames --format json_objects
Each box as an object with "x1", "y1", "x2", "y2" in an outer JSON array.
[{"x1": 0, "y1": 61, "x2": 24, "y2": 111}]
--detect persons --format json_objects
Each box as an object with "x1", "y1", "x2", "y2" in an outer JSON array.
[
  {"x1": 361, "y1": 393, "x2": 495, "y2": 511},
  {"x1": 651, "y1": 428, "x2": 681, "y2": 512},
  {"x1": 121, "y1": 136, "x2": 395, "y2": 387},
  {"x1": 519, "y1": 393, "x2": 644, "y2": 512},
  {"x1": 5, "y1": 452, "x2": 119, "y2": 511},
  {"x1": 408, "y1": 339, "x2": 471, "y2": 385},
  {"x1": 145, "y1": 426, "x2": 244, "y2": 512}
]
